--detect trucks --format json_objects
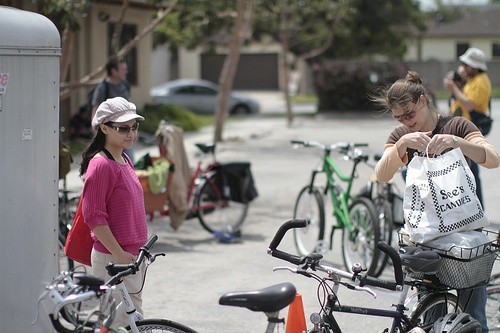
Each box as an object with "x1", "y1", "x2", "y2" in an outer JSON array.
[{"x1": 199, "y1": 47, "x2": 319, "y2": 116}]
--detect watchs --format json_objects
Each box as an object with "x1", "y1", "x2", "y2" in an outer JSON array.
[{"x1": 453, "y1": 137, "x2": 458, "y2": 143}]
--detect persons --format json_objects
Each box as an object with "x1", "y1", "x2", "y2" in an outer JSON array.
[
  {"x1": 80, "y1": 97, "x2": 148, "y2": 333},
  {"x1": 444, "y1": 47, "x2": 492, "y2": 208},
  {"x1": 92, "y1": 57, "x2": 135, "y2": 164},
  {"x1": 375, "y1": 72, "x2": 500, "y2": 333}
]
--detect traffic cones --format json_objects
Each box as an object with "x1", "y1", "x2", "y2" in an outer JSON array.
[{"x1": 285, "y1": 294, "x2": 308, "y2": 333}]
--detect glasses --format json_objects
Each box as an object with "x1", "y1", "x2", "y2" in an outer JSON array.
[
  {"x1": 105, "y1": 122, "x2": 139, "y2": 135},
  {"x1": 393, "y1": 98, "x2": 420, "y2": 123}
]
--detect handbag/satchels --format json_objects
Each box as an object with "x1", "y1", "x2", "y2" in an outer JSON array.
[
  {"x1": 469, "y1": 110, "x2": 492, "y2": 136},
  {"x1": 63, "y1": 195, "x2": 95, "y2": 267},
  {"x1": 219, "y1": 162, "x2": 258, "y2": 203},
  {"x1": 390, "y1": 182, "x2": 406, "y2": 228},
  {"x1": 402, "y1": 140, "x2": 490, "y2": 245},
  {"x1": 430, "y1": 311, "x2": 482, "y2": 333}
]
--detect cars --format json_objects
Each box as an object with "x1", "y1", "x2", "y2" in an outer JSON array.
[{"x1": 151, "y1": 78, "x2": 262, "y2": 116}]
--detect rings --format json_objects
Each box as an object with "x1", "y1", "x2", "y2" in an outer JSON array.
[{"x1": 421, "y1": 144, "x2": 423, "y2": 147}]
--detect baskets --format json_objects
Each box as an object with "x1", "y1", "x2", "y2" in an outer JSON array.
[{"x1": 397, "y1": 225, "x2": 500, "y2": 290}]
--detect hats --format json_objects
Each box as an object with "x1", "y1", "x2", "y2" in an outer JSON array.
[
  {"x1": 458, "y1": 47, "x2": 488, "y2": 73},
  {"x1": 91, "y1": 96, "x2": 145, "y2": 131}
]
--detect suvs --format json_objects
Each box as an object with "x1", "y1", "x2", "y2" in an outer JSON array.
[{"x1": 299, "y1": 48, "x2": 414, "y2": 111}]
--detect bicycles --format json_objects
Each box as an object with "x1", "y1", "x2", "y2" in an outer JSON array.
[
  {"x1": 28, "y1": 234, "x2": 295, "y2": 333},
  {"x1": 266, "y1": 139, "x2": 500, "y2": 333},
  {"x1": 149, "y1": 120, "x2": 253, "y2": 236}
]
